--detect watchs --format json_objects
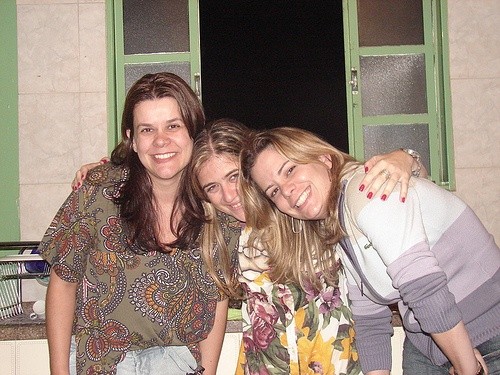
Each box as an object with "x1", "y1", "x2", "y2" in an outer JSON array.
[{"x1": 401, "y1": 148, "x2": 422, "y2": 177}]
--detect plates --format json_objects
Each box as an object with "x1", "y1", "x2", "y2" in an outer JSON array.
[{"x1": 24, "y1": 246, "x2": 51, "y2": 275}]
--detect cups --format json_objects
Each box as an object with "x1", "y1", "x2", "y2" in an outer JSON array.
[{"x1": 29, "y1": 300, "x2": 47, "y2": 321}]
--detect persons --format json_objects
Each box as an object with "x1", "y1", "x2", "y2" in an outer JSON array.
[
  {"x1": 35, "y1": 72, "x2": 243, "y2": 375},
  {"x1": 71, "y1": 119, "x2": 428, "y2": 374},
  {"x1": 238, "y1": 128, "x2": 500, "y2": 375}
]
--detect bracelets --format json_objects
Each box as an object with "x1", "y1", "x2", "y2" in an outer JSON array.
[{"x1": 453, "y1": 359, "x2": 485, "y2": 375}]
